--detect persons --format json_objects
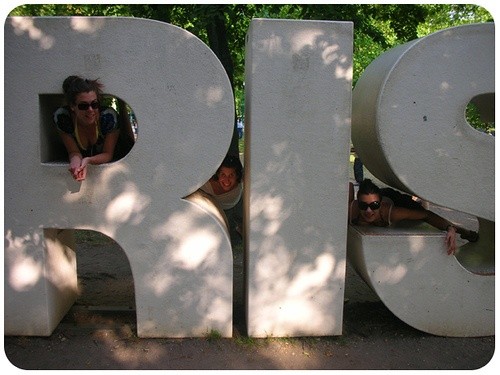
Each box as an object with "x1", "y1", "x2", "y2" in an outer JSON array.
[
  {"x1": 349, "y1": 179, "x2": 479, "y2": 255},
  {"x1": 54, "y1": 75, "x2": 120, "y2": 183},
  {"x1": 200, "y1": 156, "x2": 242, "y2": 241}
]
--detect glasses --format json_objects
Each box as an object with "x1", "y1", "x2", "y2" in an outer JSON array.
[
  {"x1": 78, "y1": 99, "x2": 100, "y2": 110},
  {"x1": 358, "y1": 200, "x2": 381, "y2": 210}
]
